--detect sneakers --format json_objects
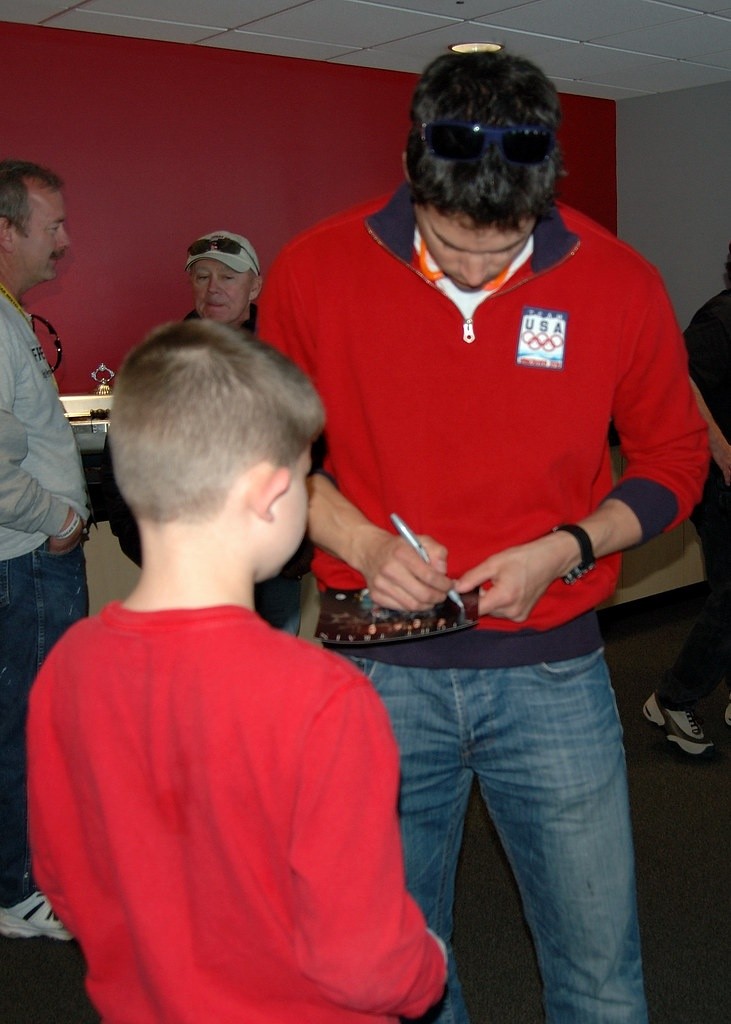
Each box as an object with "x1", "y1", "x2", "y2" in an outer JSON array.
[
  {"x1": 723, "y1": 691, "x2": 731, "y2": 727},
  {"x1": 642, "y1": 689, "x2": 716, "y2": 759},
  {"x1": 0, "y1": 889, "x2": 76, "y2": 941}
]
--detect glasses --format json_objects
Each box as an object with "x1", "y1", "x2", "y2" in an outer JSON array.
[
  {"x1": 186, "y1": 239, "x2": 260, "y2": 274},
  {"x1": 415, "y1": 122, "x2": 554, "y2": 163},
  {"x1": 30, "y1": 313, "x2": 62, "y2": 372}
]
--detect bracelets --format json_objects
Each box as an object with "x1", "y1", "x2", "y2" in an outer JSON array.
[{"x1": 55, "y1": 511, "x2": 79, "y2": 539}]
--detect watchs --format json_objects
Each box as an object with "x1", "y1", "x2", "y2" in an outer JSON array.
[{"x1": 556, "y1": 523, "x2": 595, "y2": 584}]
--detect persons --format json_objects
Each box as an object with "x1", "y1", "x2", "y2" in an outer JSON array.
[
  {"x1": 111, "y1": 232, "x2": 313, "y2": 638},
  {"x1": 259, "y1": 53, "x2": 707, "y2": 1024},
  {"x1": 642, "y1": 245, "x2": 731, "y2": 758},
  {"x1": 0, "y1": 152, "x2": 95, "y2": 944},
  {"x1": 29, "y1": 322, "x2": 453, "y2": 1024}
]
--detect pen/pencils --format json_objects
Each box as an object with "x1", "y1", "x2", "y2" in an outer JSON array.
[{"x1": 391, "y1": 512, "x2": 466, "y2": 608}]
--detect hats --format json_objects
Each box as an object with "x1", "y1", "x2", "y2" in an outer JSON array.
[{"x1": 184, "y1": 230, "x2": 260, "y2": 276}]
